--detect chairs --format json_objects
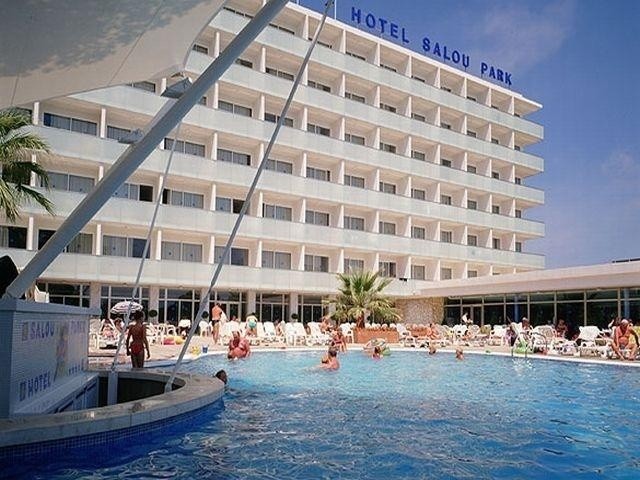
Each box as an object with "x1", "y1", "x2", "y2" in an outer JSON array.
[
  {"x1": 197, "y1": 317, "x2": 639, "y2": 359},
  {"x1": 91, "y1": 318, "x2": 191, "y2": 350}
]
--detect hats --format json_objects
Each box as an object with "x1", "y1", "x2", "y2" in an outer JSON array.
[{"x1": 621, "y1": 319, "x2": 628, "y2": 324}]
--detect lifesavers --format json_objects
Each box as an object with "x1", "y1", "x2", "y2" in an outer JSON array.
[{"x1": 364, "y1": 337, "x2": 388, "y2": 352}]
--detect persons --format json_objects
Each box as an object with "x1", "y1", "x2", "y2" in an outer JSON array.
[
  {"x1": 100, "y1": 310, "x2": 150, "y2": 368},
  {"x1": 357, "y1": 312, "x2": 568, "y2": 361},
  {"x1": 216, "y1": 370, "x2": 227, "y2": 384},
  {"x1": 571, "y1": 317, "x2": 640, "y2": 361},
  {"x1": 180, "y1": 303, "x2": 347, "y2": 369}
]
